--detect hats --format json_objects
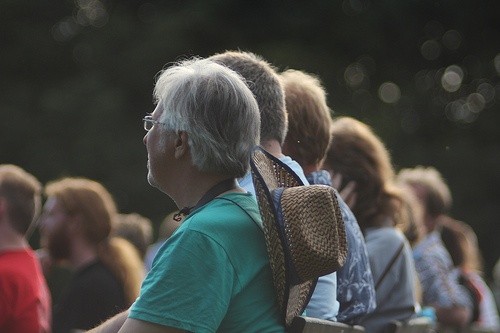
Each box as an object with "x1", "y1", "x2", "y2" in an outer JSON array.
[{"x1": 249, "y1": 146, "x2": 348, "y2": 326}]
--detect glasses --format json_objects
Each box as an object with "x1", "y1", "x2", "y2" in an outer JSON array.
[{"x1": 142, "y1": 115, "x2": 179, "y2": 133}]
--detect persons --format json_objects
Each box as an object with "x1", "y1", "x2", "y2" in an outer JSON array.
[
  {"x1": 40, "y1": 177, "x2": 128, "y2": 333},
  {"x1": 0, "y1": 163, "x2": 54, "y2": 333},
  {"x1": 0, "y1": 47, "x2": 500, "y2": 333},
  {"x1": 85, "y1": 59, "x2": 288, "y2": 333}
]
